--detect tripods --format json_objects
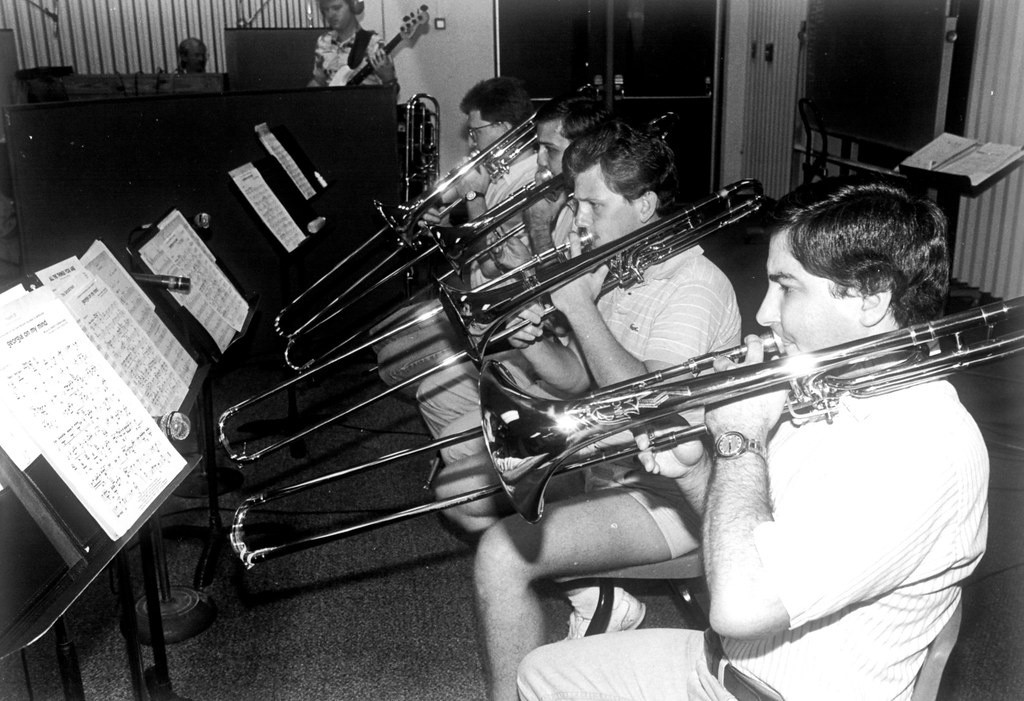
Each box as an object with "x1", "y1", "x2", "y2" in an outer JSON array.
[{"x1": 126, "y1": 207, "x2": 276, "y2": 589}]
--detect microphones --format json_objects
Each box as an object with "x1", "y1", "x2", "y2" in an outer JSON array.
[
  {"x1": 140, "y1": 213, "x2": 212, "y2": 230},
  {"x1": 130, "y1": 273, "x2": 190, "y2": 289}
]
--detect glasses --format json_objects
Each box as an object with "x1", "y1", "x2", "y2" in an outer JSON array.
[{"x1": 468, "y1": 119, "x2": 504, "y2": 141}]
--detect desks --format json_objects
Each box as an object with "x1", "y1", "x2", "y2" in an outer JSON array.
[{"x1": 1, "y1": 79, "x2": 399, "y2": 284}]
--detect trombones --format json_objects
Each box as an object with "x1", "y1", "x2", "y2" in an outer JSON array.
[
  {"x1": 218, "y1": 177, "x2": 766, "y2": 463},
  {"x1": 272, "y1": 105, "x2": 548, "y2": 339},
  {"x1": 226, "y1": 295, "x2": 1024, "y2": 572},
  {"x1": 282, "y1": 109, "x2": 681, "y2": 372}
]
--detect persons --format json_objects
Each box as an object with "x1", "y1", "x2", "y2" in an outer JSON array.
[
  {"x1": 434, "y1": 115, "x2": 742, "y2": 701},
  {"x1": 370, "y1": 75, "x2": 537, "y2": 393},
  {"x1": 180, "y1": 37, "x2": 207, "y2": 73},
  {"x1": 414, "y1": 92, "x2": 618, "y2": 466},
  {"x1": 515, "y1": 172, "x2": 991, "y2": 701},
  {"x1": 307, "y1": 0, "x2": 400, "y2": 95}
]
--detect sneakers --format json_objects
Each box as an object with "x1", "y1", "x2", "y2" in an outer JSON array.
[{"x1": 567, "y1": 590, "x2": 647, "y2": 642}]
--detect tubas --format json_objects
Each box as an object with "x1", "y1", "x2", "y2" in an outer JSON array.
[{"x1": 403, "y1": 92, "x2": 441, "y2": 225}]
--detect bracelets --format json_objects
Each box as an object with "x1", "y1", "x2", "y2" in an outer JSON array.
[{"x1": 383, "y1": 78, "x2": 400, "y2": 94}]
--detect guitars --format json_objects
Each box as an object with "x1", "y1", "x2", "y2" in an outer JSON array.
[{"x1": 326, "y1": 3, "x2": 431, "y2": 87}]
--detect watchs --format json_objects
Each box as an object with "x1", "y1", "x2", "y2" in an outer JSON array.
[
  {"x1": 712, "y1": 431, "x2": 768, "y2": 463},
  {"x1": 463, "y1": 191, "x2": 485, "y2": 202}
]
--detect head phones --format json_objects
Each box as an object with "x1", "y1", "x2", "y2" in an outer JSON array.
[{"x1": 348, "y1": 0, "x2": 365, "y2": 16}]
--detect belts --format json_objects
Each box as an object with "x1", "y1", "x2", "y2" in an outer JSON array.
[{"x1": 701, "y1": 626, "x2": 776, "y2": 701}]
